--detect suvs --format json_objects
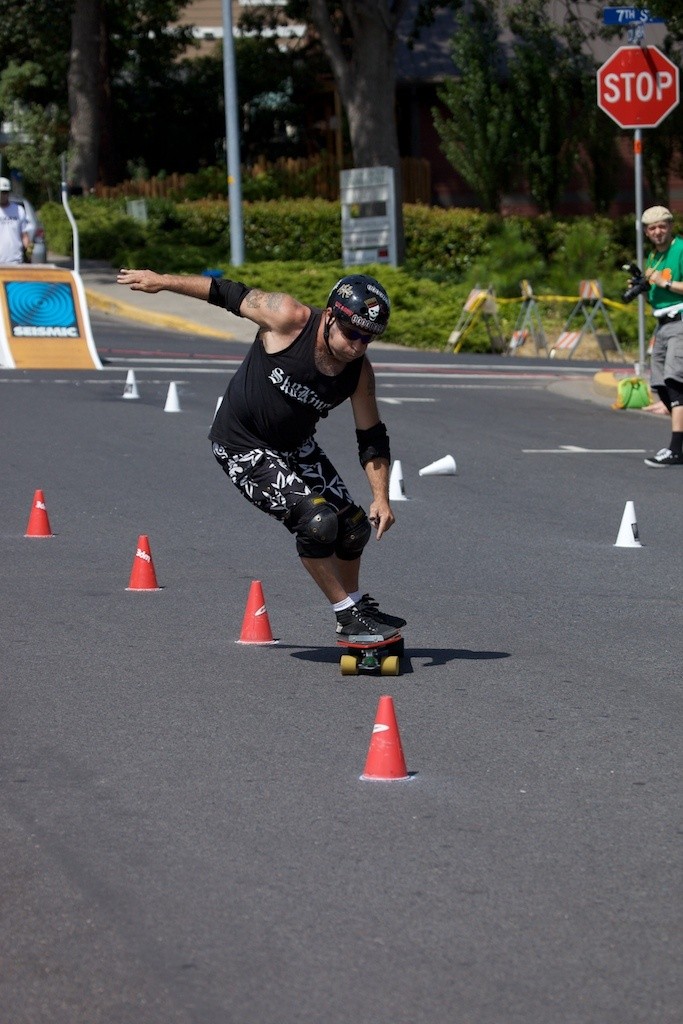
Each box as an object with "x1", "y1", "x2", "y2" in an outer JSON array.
[{"x1": 3, "y1": 200, "x2": 50, "y2": 265}]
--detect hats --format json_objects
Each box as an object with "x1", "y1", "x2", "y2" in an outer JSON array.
[
  {"x1": 642, "y1": 206, "x2": 673, "y2": 225},
  {"x1": 0, "y1": 177, "x2": 11, "y2": 193}
]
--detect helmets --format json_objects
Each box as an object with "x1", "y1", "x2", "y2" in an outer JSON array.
[{"x1": 327, "y1": 272, "x2": 390, "y2": 335}]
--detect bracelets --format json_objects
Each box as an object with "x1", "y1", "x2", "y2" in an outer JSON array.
[{"x1": 664, "y1": 281, "x2": 671, "y2": 290}]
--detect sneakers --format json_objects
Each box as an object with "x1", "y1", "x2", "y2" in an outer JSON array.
[
  {"x1": 336, "y1": 606, "x2": 401, "y2": 645},
  {"x1": 355, "y1": 593, "x2": 406, "y2": 629},
  {"x1": 644, "y1": 448, "x2": 683, "y2": 468}
]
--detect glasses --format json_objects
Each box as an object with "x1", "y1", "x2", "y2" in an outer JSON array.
[{"x1": 334, "y1": 318, "x2": 375, "y2": 345}]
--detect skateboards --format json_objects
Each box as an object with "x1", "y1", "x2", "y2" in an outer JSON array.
[{"x1": 336, "y1": 632, "x2": 403, "y2": 676}]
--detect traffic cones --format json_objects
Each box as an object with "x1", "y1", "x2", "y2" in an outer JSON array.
[
  {"x1": 164, "y1": 381, "x2": 182, "y2": 412},
  {"x1": 389, "y1": 458, "x2": 409, "y2": 502},
  {"x1": 115, "y1": 369, "x2": 142, "y2": 398},
  {"x1": 124, "y1": 534, "x2": 164, "y2": 590},
  {"x1": 418, "y1": 453, "x2": 459, "y2": 478},
  {"x1": 355, "y1": 694, "x2": 411, "y2": 781},
  {"x1": 22, "y1": 492, "x2": 56, "y2": 538},
  {"x1": 210, "y1": 396, "x2": 223, "y2": 429},
  {"x1": 614, "y1": 502, "x2": 645, "y2": 547},
  {"x1": 236, "y1": 580, "x2": 279, "y2": 643}
]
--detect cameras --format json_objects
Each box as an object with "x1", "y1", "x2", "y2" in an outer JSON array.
[{"x1": 620, "y1": 263, "x2": 652, "y2": 303}]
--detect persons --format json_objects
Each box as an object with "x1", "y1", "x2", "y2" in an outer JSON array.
[
  {"x1": 117, "y1": 269, "x2": 406, "y2": 642},
  {"x1": 627, "y1": 205, "x2": 683, "y2": 467},
  {"x1": 0, "y1": 178, "x2": 31, "y2": 263}
]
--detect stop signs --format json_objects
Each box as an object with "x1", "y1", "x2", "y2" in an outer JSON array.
[{"x1": 597, "y1": 44, "x2": 680, "y2": 128}]
